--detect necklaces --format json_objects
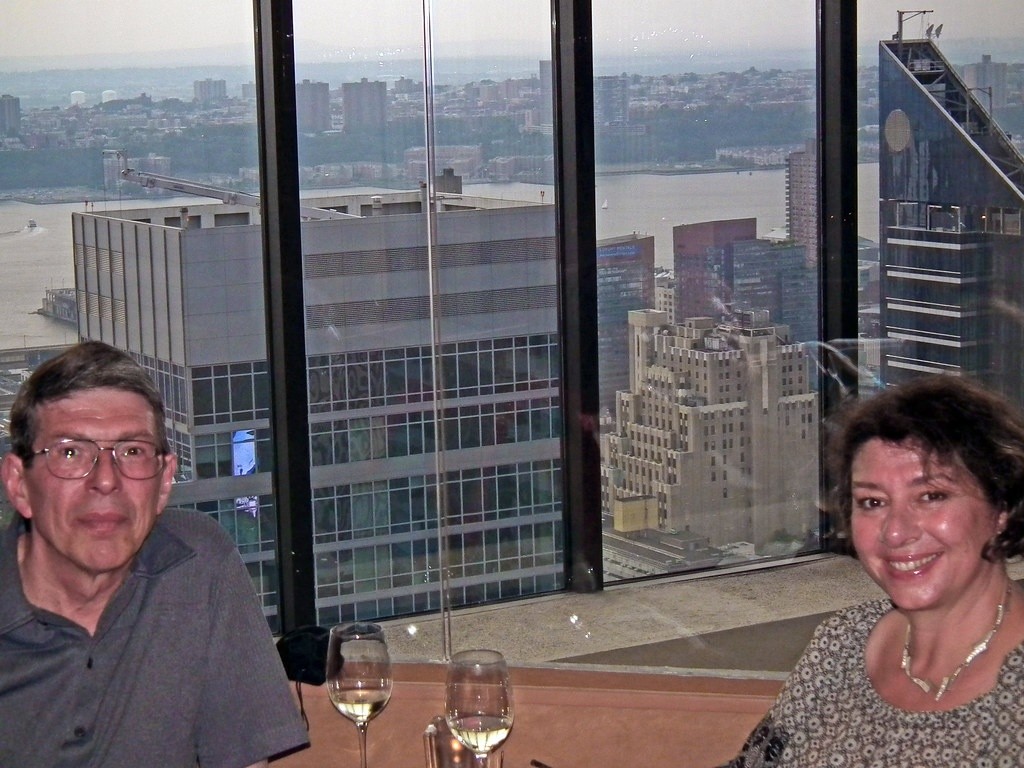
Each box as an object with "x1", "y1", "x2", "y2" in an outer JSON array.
[{"x1": 900, "y1": 576, "x2": 1013, "y2": 701}]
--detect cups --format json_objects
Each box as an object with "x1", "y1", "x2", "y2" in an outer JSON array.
[{"x1": 423, "y1": 714, "x2": 501, "y2": 768}]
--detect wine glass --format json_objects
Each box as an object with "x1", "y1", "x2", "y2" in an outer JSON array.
[
  {"x1": 444, "y1": 647, "x2": 515, "y2": 768},
  {"x1": 326, "y1": 622, "x2": 393, "y2": 767}
]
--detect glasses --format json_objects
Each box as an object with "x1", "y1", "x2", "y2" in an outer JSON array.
[{"x1": 20, "y1": 438, "x2": 170, "y2": 480}]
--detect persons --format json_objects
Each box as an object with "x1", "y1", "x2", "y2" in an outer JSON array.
[
  {"x1": 0, "y1": 339, "x2": 311, "y2": 768},
  {"x1": 715, "y1": 373, "x2": 1024, "y2": 768}
]
